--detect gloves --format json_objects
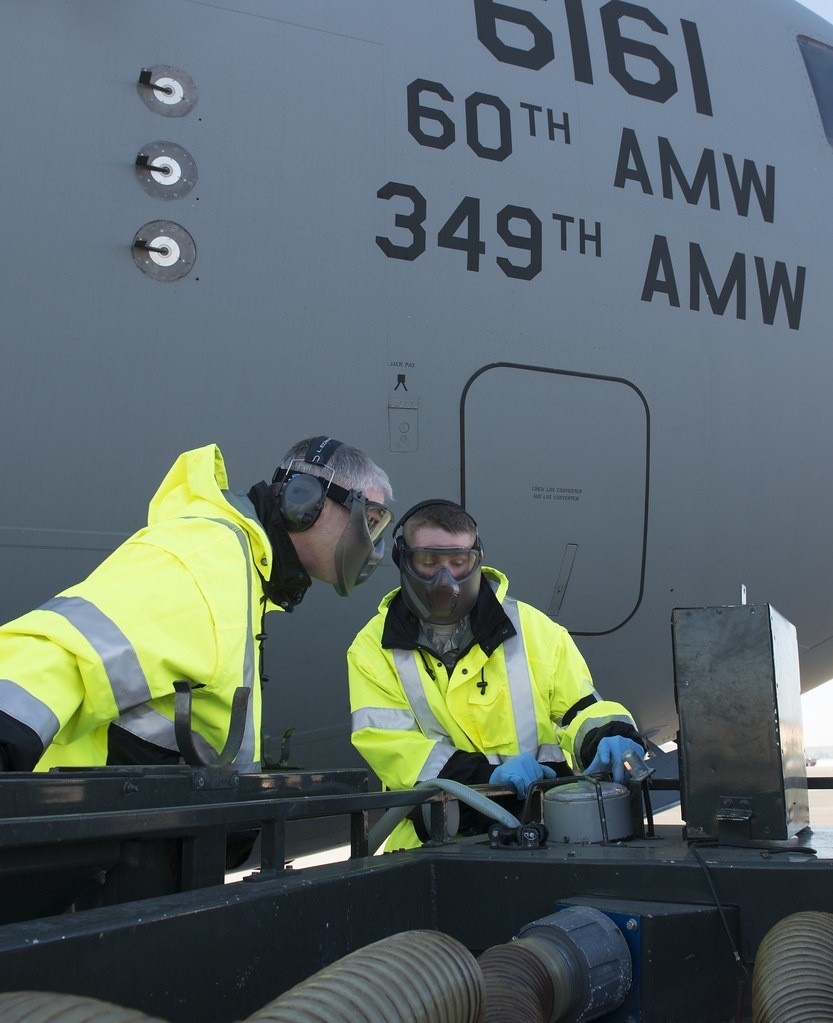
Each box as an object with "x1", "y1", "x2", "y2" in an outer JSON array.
[
  {"x1": 489, "y1": 752, "x2": 557, "y2": 802},
  {"x1": 576, "y1": 734, "x2": 649, "y2": 783}
]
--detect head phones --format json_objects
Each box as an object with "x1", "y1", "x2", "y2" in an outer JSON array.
[
  {"x1": 392, "y1": 499, "x2": 485, "y2": 569},
  {"x1": 276, "y1": 436, "x2": 343, "y2": 533}
]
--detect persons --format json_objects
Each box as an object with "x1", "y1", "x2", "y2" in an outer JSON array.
[
  {"x1": 0, "y1": 435, "x2": 393, "y2": 929},
  {"x1": 346, "y1": 499, "x2": 648, "y2": 853}
]
